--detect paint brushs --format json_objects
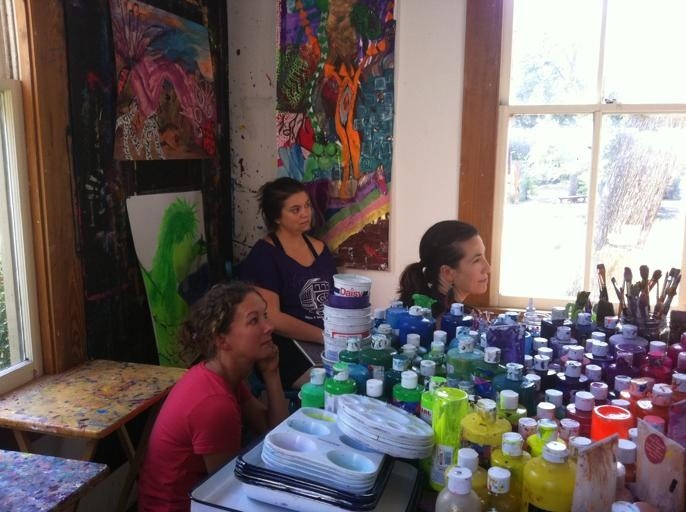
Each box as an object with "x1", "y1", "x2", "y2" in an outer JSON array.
[{"x1": 597, "y1": 264, "x2": 682, "y2": 324}]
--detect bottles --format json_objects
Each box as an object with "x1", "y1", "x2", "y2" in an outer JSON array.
[{"x1": 299, "y1": 298, "x2": 686, "y2": 512}]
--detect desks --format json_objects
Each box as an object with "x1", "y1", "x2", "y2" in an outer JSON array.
[
  {"x1": 0, "y1": 359, "x2": 191, "y2": 512},
  {"x1": 0, "y1": 448, "x2": 112, "y2": 512}
]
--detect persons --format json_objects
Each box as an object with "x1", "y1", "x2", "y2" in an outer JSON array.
[
  {"x1": 242, "y1": 176, "x2": 339, "y2": 387},
  {"x1": 137, "y1": 282, "x2": 289, "y2": 512},
  {"x1": 395, "y1": 219, "x2": 492, "y2": 330}
]
